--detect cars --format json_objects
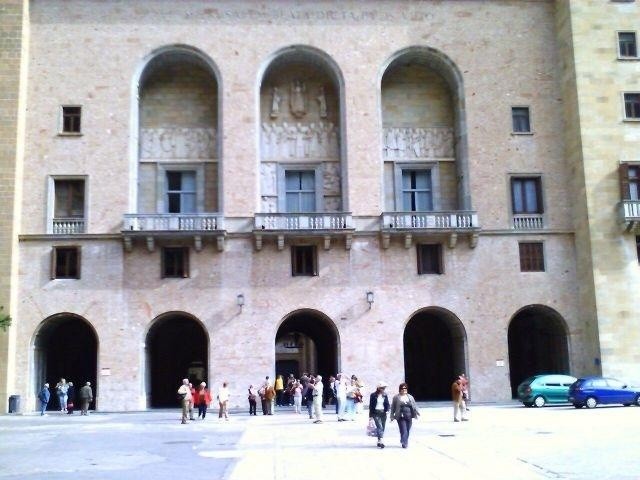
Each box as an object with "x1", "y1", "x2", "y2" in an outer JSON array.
[
  {"x1": 567, "y1": 377, "x2": 640, "y2": 410},
  {"x1": 515, "y1": 373, "x2": 581, "y2": 409}
]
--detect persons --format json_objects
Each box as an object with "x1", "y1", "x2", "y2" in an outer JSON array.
[
  {"x1": 54, "y1": 378, "x2": 69, "y2": 412},
  {"x1": 317, "y1": 85, "x2": 327, "y2": 117},
  {"x1": 273, "y1": 86, "x2": 280, "y2": 113},
  {"x1": 391, "y1": 383, "x2": 420, "y2": 448},
  {"x1": 66, "y1": 381, "x2": 74, "y2": 414},
  {"x1": 77, "y1": 380, "x2": 94, "y2": 416},
  {"x1": 451, "y1": 376, "x2": 468, "y2": 422},
  {"x1": 292, "y1": 78, "x2": 306, "y2": 113},
  {"x1": 460, "y1": 374, "x2": 468, "y2": 401},
  {"x1": 178, "y1": 373, "x2": 363, "y2": 424},
  {"x1": 39, "y1": 383, "x2": 51, "y2": 415},
  {"x1": 368, "y1": 383, "x2": 391, "y2": 449}
]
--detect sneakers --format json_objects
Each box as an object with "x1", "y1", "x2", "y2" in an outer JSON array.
[
  {"x1": 376, "y1": 442, "x2": 386, "y2": 449},
  {"x1": 178, "y1": 409, "x2": 364, "y2": 425},
  {"x1": 401, "y1": 442, "x2": 409, "y2": 448},
  {"x1": 454, "y1": 418, "x2": 459, "y2": 422},
  {"x1": 40, "y1": 409, "x2": 89, "y2": 418},
  {"x1": 461, "y1": 418, "x2": 469, "y2": 421}
]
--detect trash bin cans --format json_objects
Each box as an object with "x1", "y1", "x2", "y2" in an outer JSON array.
[{"x1": 9, "y1": 394, "x2": 20, "y2": 413}]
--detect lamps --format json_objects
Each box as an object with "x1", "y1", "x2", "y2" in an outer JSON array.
[
  {"x1": 366, "y1": 291, "x2": 374, "y2": 310},
  {"x1": 235, "y1": 294, "x2": 245, "y2": 313}
]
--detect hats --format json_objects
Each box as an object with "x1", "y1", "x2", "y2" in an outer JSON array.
[{"x1": 375, "y1": 381, "x2": 389, "y2": 389}]
